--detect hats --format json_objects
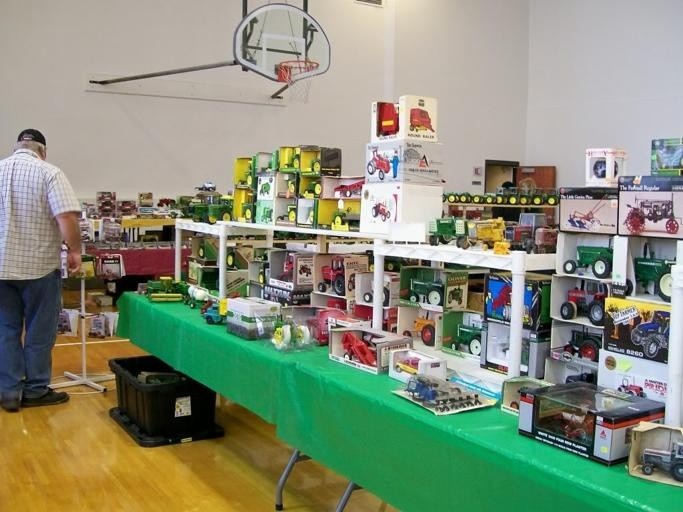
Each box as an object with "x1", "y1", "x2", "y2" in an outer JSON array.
[{"x1": 17, "y1": 128, "x2": 46, "y2": 146}]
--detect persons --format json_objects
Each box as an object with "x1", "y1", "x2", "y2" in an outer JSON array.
[
  {"x1": 491, "y1": 181, "x2": 521, "y2": 221},
  {"x1": 519, "y1": 178, "x2": 545, "y2": 213},
  {"x1": 0, "y1": 128, "x2": 83, "y2": 412},
  {"x1": 388, "y1": 150, "x2": 399, "y2": 178}
]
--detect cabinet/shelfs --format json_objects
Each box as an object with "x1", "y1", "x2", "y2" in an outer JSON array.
[{"x1": 176, "y1": 203, "x2": 682, "y2": 394}]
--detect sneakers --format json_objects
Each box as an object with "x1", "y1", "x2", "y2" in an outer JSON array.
[{"x1": 1, "y1": 389, "x2": 69, "y2": 412}]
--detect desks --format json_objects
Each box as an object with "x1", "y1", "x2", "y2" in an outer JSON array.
[{"x1": 45, "y1": 246, "x2": 683, "y2": 512}]
